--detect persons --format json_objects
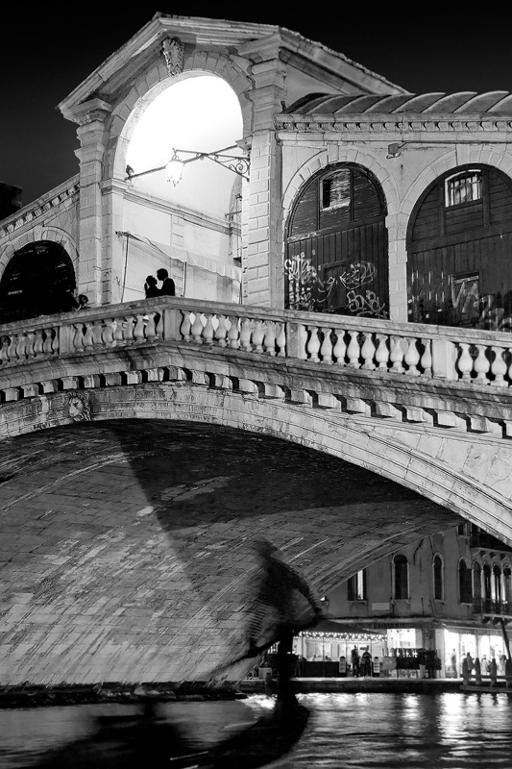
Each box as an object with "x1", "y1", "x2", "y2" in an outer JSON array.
[
  {"x1": 248, "y1": 539, "x2": 322, "y2": 707},
  {"x1": 489, "y1": 658, "x2": 498, "y2": 689},
  {"x1": 78, "y1": 293, "x2": 91, "y2": 308},
  {"x1": 157, "y1": 267, "x2": 176, "y2": 294},
  {"x1": 450, "y1": 649, "x2": 458, "y2": 677},
  {"x1": 472, "y1": 657, "x2": 483, "y2": 685},
  {"x1": 462, "y1": 651, "x2": 472, "y2": 686},
  {"x1": 466, "y1": 652, "x2": 473, "y2": 681},
  {"x1": 352, "y1": 648, "x2": 361, "y2": 677},
  {"x1": 361, "y1": 648, "x2": 372, "y2": 678},
  {"x1": 55, "y1": 293, "x2": 79, "y2": 313},
  {"x1": 505, "y1": 657, "x2": 512, "y2": 689},
  {"x1": 143, "y1": 275, "x2": 159, "y2": 298}
]
